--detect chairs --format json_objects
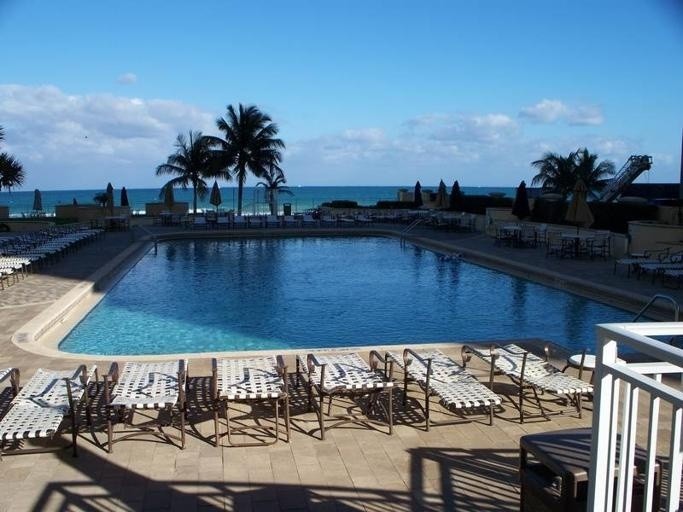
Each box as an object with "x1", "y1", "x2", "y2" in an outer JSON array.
[
  {"x1": 494, "y1": 219, "x2": 683, "y2": 289},
  {"x1": 192, "y1": 214, "x2": 373, "y2": 231},
  {"x1": 0, "y1": 222, "x2": 106, "y2": 290}
]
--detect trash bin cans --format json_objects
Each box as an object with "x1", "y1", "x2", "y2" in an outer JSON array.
[
  {"x1": 520, "y1": 427, "x2": 662, "y2": 512},
  {"x1": 284, "y1": 203, "x2": 291, "y2": 216}
]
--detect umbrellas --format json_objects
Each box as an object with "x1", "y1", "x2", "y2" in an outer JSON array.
[
  {"x1": 210, "y1": 180, "x2": 222, "y2": 218},
  {"x1": 33, "y1": 188, "x2": 43, "y2": 217},
  {"x1": 512, "y1": 180, "x2": 530, "y2": 223},
  {"x1": 565, "y1": 182, "x2": 593, "y2": 234},
  {"x1": 164, "y1": 182, "x2": 175, "y2": 212}
]
